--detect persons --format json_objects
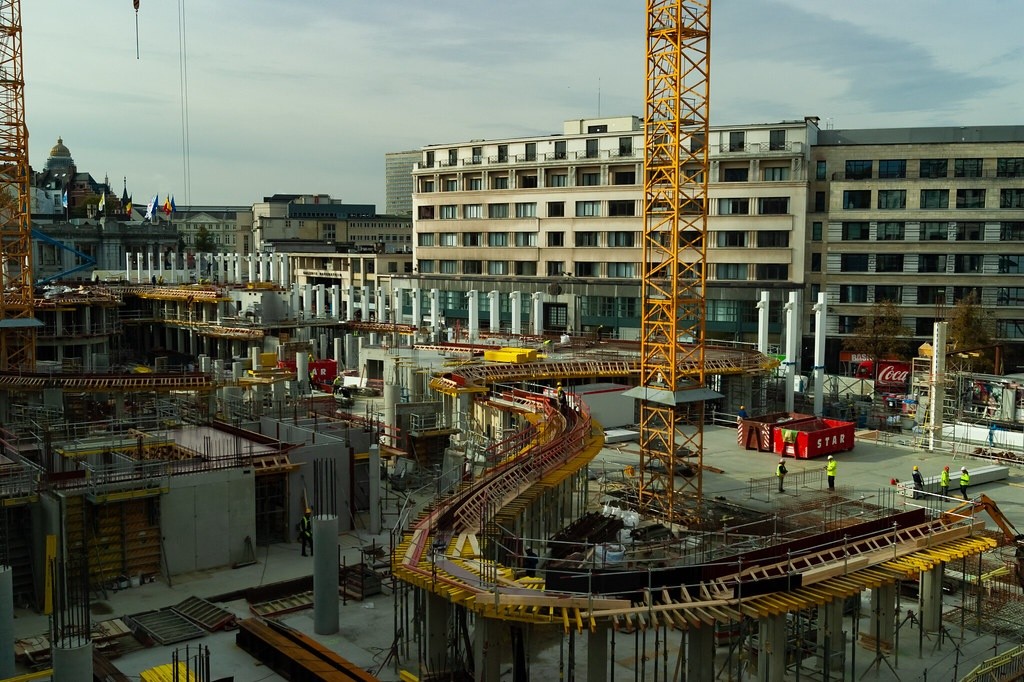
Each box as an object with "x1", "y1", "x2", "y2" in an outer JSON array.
[
  {"x1": 556, "y1": 382, "x2": 567, "y2": 415},
  {"x1": 308, "y1": 354, "x2": 314, "y2": 362},
  {"x1": 301, "y1": 509, "x2": 313, "y2": 557},
  {"x1": 777, "y1": 459, "x2": 788, "y2": 492},
  {"x1": 332, "y1": 376, "x2": 341, "y2": 395},
  {"x1": 739, "y1": 406, "x2": 748, "y2": 419},
  {"x1": 157, "y1": 275, "x2": 164, "y2": 285},
  {"x1": 912, "y1": 466, "x2": 925, "y2": 499},
  {"x1": 959, "y1": 467, "x2": 970, "y2": 500},
  {"x1": 152, "y1": 275, "x2": 156, "y2": 288},
  {"x1": 939, "y1": 466, "x2": 951, "y2": 502},
  {"x1": 824, "y1": 455, "x2": 837, "y2": 491},
  {"x1": 352, "y1": 327, "x2": 360, "y2": 336}
]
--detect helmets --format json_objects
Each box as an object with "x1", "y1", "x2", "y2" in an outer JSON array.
[
  {"x1": 944, "y1": 466, "x2": 949, "y2": 470},
  {"x1": 961, "y1": 467, "x2": 966, "y2": 470},
  {"x1": 740, "y1": 406, "x2": 745, "y2": 409},
  {"x1": 305, "y1": 509, "x2": 312, "y2": 513},
  {"x1": 913, "y1": 466, "x2": 918, "y2": 470},
  {"x1": 828, "y1": 456, "x2": 833, "y2": 459},
  {"x1": 780, "y1": 458, "x2": 786, "y2": 462},
  {"x1": 558, "y1": 387, "x2": 563, "y2": 391},
  {"x1": 556, "y1": 381, "x2": 562, "y2": 385}
]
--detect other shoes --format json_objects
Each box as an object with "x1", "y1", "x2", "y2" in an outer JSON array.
[
  {"x1": 302, "y1": 554, "x2": 308, "y2": 557},
  {"x1": 945, "y1": 500, "x2": 950, "y2": 502},
  {"x1": 916, "y1": 498, "x2": 919, "y2": 500},
  {"x1": 779, "y1": 489, "x2": 785, "y2": 491},
  {"x1": 939, "y1": 499, "x2": 943, "y2": 501}
]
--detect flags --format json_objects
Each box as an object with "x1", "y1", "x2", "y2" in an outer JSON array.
[
  {"x1": 164, "y1": 196, "x2": 176, "y2": 215},
  {"x1": 145, "y1": 195, "x2": 158, "y2": 219},
  {"x1": 62, "y1": 191, "x2": 68, "y2": 208},
  {"x1": 124, "y1": 196, "x2": 132, "y2": 214},
  {"x1": 98, "y1": 193, "x2": 105, "y2": 211}
]
